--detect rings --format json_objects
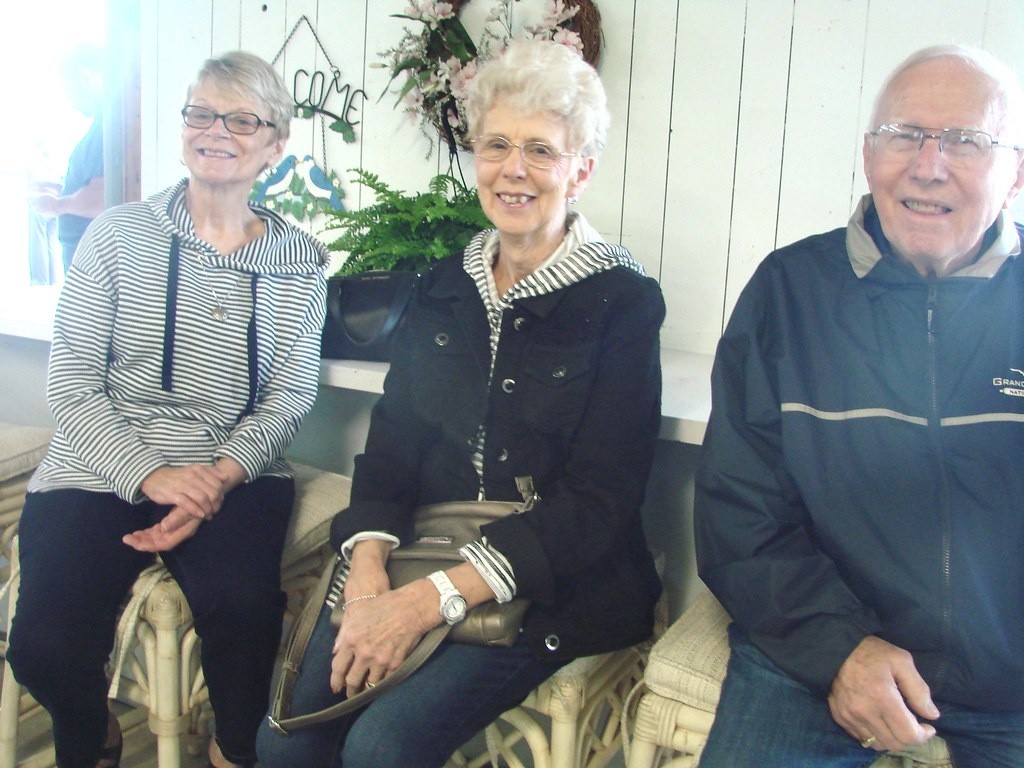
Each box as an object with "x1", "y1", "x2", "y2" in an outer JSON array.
[{"x1": 862, "y1": 738, "x2": 872, "y2": 748}]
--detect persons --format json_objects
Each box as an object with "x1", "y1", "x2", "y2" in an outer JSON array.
[
  {"x1": 693, "y1": 45, "x2": 1024, "y2": 768},
  {"x1": 5, "y1": 53, "x2": 329, "y2": 768},
  {"x1": 25, "y1": 55, "x2": 104, "y2": 287},
  {"x1": 254, "y1": 44, "x2": 665, "y2": 768}
]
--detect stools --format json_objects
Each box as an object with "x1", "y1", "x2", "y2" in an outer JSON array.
[{"x1": 0, "y1": 420, "x2": 952, "y2": 768}]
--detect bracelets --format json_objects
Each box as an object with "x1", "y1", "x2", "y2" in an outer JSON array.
[{"x1": 342, "y1": 595, "x2": 376, "y2": 612}]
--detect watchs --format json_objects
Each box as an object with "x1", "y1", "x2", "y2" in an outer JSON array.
[{"x1": 426, "y1": 569, "x2": 467, "y2": 625}]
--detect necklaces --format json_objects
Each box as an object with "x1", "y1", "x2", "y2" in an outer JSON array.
[{"x1": 195, "y1": 249, "x2": 244, "y2": 321}]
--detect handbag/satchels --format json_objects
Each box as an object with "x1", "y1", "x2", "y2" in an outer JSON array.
[{"x1": 263, "y1": 500, "x2": 537, "y2": 731}]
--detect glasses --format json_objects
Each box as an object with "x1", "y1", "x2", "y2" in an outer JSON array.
[
  {"x1": 180, "y1": 88, "x2": 278, "y2": 135},
  {"x1": 870, "y1": 122, "x2": 1020, "y2": 162},
  {"x1": 462, "y1": 135, "x2": 584, "y2": 170}
]
